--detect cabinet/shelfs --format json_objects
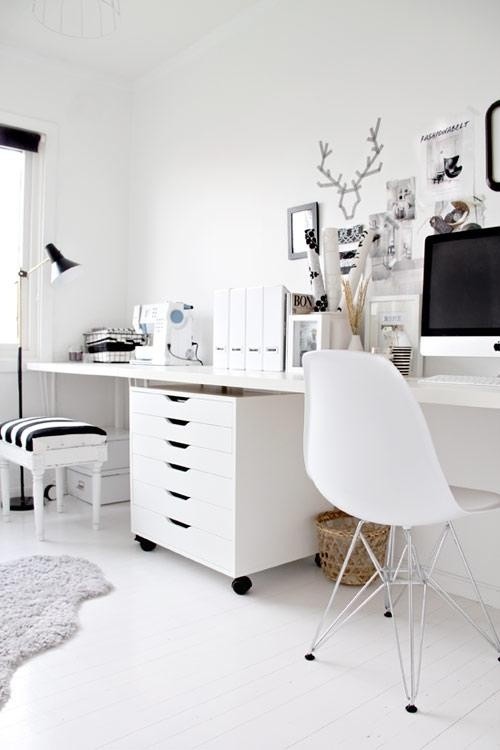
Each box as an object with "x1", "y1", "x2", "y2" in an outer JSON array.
[
  {"x1": 64, "y1": 428, "x2": 130, "y2": 505},
  {"x1": 124, "y1": 386, "x2": 327, "y2": 595}
]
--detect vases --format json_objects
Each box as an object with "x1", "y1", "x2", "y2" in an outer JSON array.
[{"x1": 346, "y1": 333, "x2": 364, "y2": 352}]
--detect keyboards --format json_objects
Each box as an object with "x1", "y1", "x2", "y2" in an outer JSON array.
[{"x1": 420, "y1": 375, "x2": 500, "y2": 386}]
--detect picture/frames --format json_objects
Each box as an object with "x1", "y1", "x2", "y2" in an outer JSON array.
[
  {"x1": 366, "y1": 295, "x2": 423, "y2": 356},
  {"x1": 286, "y1": 313, "x2": 327, "y2": 376},
  {"x1": 284, "y1": 200, "x2": 320, "y2": 260}
]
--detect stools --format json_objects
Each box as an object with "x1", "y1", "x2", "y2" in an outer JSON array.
[{"x1": 0, "y1": 417, "x2": 107, "y2": 544}]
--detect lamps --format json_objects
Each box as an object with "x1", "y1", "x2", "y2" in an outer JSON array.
[{"x1": 9, "y1": 242, "x2": 82, "y2": 510}]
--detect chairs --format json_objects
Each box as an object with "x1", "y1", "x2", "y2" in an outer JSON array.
[{"x1": 302, "y1": 350, "x2": 500, "y2": 714}]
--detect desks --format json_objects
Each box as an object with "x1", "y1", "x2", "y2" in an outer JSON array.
[{"x1": 27, "y1": 352, "x2": 500, "y2": 495}]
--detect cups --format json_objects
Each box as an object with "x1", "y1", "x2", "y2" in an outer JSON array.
[{"x1": 391, "y1": 346, "x2": 414, "y2": 377}]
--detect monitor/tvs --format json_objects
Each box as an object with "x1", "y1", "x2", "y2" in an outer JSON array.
[{"x1": 418, "y1": 224, "x2": 500, "y2": 340}]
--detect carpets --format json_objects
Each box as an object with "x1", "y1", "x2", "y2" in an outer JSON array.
[{"x1": 0, "y1": 552, "x2": 114, "y2": 714}]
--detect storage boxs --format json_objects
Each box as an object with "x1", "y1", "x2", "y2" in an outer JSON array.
[{"x1": 83, "y1": 332, "x2": 138, "y2": 364}]
